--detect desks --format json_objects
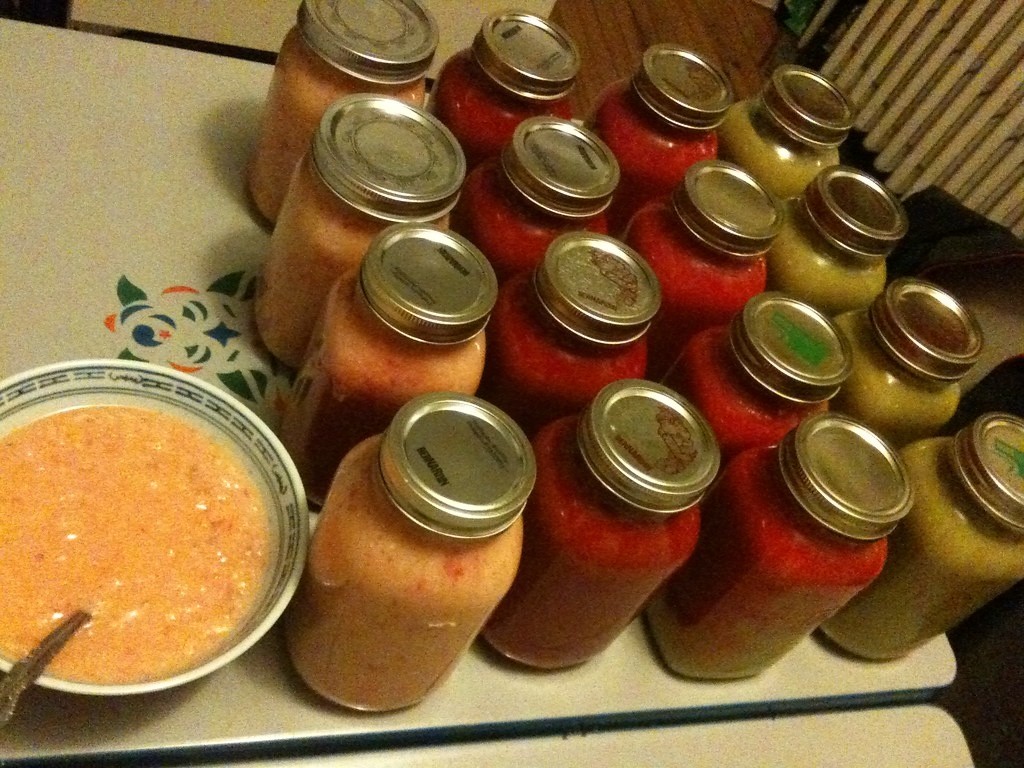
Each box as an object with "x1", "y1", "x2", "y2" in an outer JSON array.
[{"x1": 0, "y1": 19, "x2": 974, "y2": 768}]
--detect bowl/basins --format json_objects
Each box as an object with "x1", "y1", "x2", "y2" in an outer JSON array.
[{"x1": 0, "y1": 358, "x2": 309, "y2": 695}]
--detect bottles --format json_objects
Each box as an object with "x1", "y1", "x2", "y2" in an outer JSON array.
[
  {"x1": 251, "y1": 92, "x2": 621, "y2": 353},
  {"x1": 480, "y1": 377, "x2": 722, "y2": 669},
  {"x1": 644, "y1": 412, "x2": 917, "y2": 681},
  {"x1": 267, "y1": 222, "x2": 663, "y2": 506},
  {"x1": 820, "y1": 411, "x2": 1024, "y2": 659},
  {"x1": 624, "y1": 160, "x2": 909, "y2": 378},
  {"x1": 585, "y1": 42, "x2": 858, "y2": 233},
  {"x1": 248, "y1": 2, "x2": 581, "y2": 226},
  {"x1": 662, "y1": 278, "x2": 984, "y2": 465},
  {"x1": 278, "y1": 390, "x2": 537, "y2": 712}
]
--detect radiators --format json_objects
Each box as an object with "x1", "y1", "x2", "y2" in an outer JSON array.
[{"x1": 817, "y1": 0, "x2": 1024, "y2": 242}]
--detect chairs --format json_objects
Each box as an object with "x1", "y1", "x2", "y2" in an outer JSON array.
[{"x1": 67, "y1": 0, "x2": 556, "y2": 82}]
